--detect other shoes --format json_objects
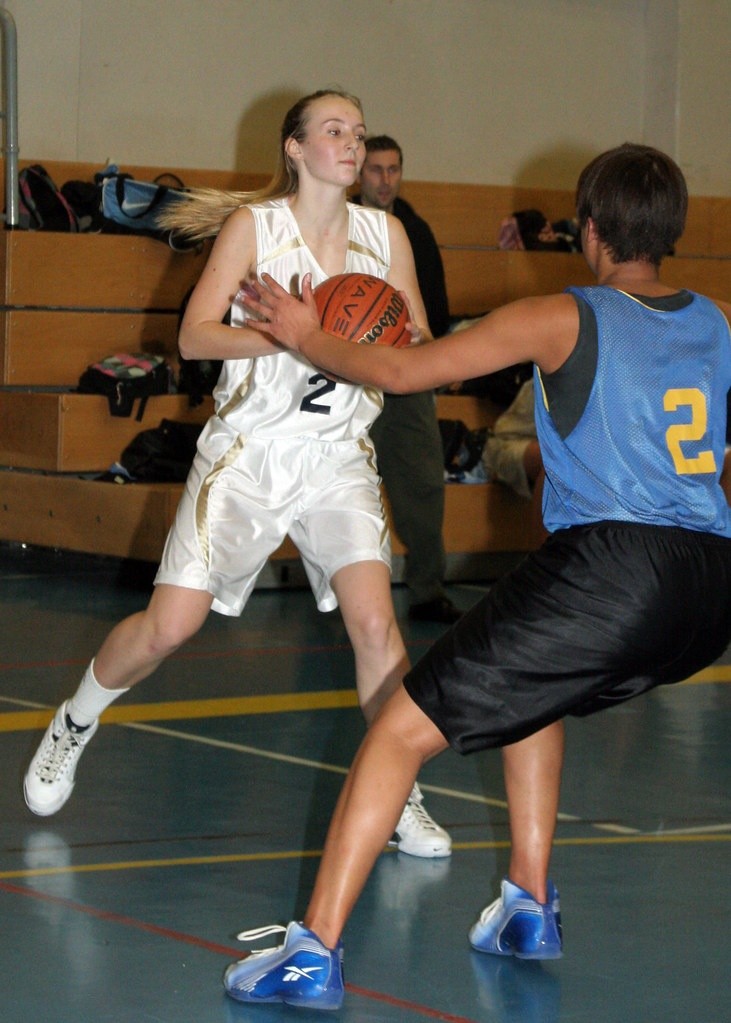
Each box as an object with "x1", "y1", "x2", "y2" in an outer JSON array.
[{"x1": 405, "y1": 596, "x2": 467, "y2": 621}]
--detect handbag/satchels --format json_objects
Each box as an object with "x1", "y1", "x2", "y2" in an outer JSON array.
[{"x1": 102, "y1": 170, "x2": 195, "y2": 239}]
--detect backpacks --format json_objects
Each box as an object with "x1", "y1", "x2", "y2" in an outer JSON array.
[
  {"x1": 85, "y1": 351, "x2": 171, "y2": 421},
  {"x1": 16, "y1": 164, "x2": 77, "y2": 232}
]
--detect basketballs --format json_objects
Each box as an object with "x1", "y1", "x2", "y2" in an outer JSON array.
[{"x1": 297, "y1": 270, "x2": 413, "y2": 387}]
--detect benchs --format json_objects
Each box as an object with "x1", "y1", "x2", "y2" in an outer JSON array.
[{"x1": 1, "y1": 158, "x2": 730, "y2": 598}]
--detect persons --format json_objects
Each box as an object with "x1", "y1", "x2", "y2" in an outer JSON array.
[
  {"x1": 349, "y1": 137, "x2": 466, "y2": 616},
  {"x1": 222, "y1": 141, "x2": 731, "y2": 1010},
  {"x1": 482, "y1": 378, "x2": 545, "y2": 539},
  {"x1": 22, "y1": 88, "x2": 453, "y2": 860}
]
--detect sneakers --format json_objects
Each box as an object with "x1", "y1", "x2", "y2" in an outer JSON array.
[
  {"x1": 222, "y1": 921, "x2": 345, "y2": 1011},
  {"x1": 467, "y1": 879, "x2": 562, "y2": 959},
  {"x1": 23, "y1": 699, "x2": 100, "y2": 816},
  {"x1": 387, "y1": 784, "x2": 450, "y2": 858}
]
